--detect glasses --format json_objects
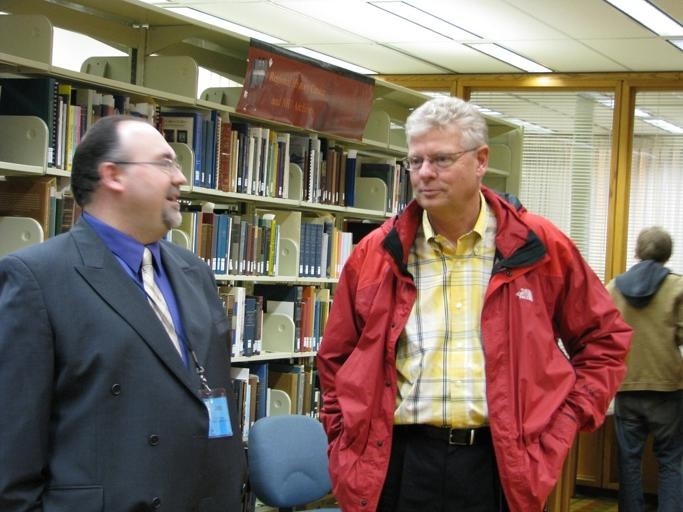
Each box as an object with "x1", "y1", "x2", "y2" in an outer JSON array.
[
  {"x1": 115, "y1": 159, "x2": 181, "y2": 174},
  {"x1": 404, "y1": 146, "x2": 478, "y2": 171}
]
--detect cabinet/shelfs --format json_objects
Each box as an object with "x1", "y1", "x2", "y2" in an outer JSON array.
[{"x1": 0, "y1": 0, "x2": 525, "y2": 448}]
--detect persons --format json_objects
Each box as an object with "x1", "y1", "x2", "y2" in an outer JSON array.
[
  {"x1": 317, "y1": 95, "x2": 635, "y2": 511},
  {"x1": 603, "y1": 224, "x2": 682, "y2": 510},
  {"x1": 1, "y1": 112, "x2": 248, "y2": 510}
]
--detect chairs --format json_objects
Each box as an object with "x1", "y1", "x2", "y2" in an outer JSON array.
[{"x1": 248, "y1": 416, "x2": 341, "y2": 512}]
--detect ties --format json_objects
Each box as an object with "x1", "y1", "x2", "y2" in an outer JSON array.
[{"x1": 140, "y1": 246, "x2": 182, "y2": 356}]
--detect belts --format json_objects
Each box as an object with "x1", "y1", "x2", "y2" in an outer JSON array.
[{"x1": 394, "y1": 425, "x2": 492, "y2": 446}]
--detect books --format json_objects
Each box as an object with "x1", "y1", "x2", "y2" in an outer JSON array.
[{"x1": 0, "y1": 72, "x2": 415, "y2": 443}]
